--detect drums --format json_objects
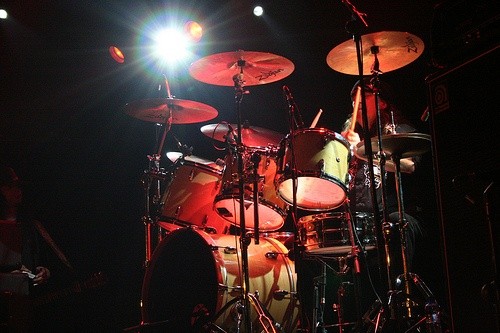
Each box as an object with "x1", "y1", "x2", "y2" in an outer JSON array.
[
  {"x1": 153, "y1": 157, "x2": 232, "y2": 236},
  {"x1": 212, "y1": 147, "x2": 288, "y2": 233},
  {"x1": 296, "y1": 210, "x2": 377, "y2": 255},
  {"x1": 139, "y1": 222, "x2": 301, "y2": 333},
  {"x1": 350, "y1": 67, "x2": 437, "y2": 160},
  {"x1": 245, "y1": 230, "x2": 294, "y2": 244},
  {"x1": 274, "y1": 126, "x2": 350, "y2": 212}
]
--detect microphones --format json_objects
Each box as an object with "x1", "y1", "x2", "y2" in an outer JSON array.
[{"x1": 283, "y1": 86, "x2": 304, "y2": 129}]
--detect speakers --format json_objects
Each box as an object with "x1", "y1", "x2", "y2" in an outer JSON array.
[{"x1": 427, "y1": 46, "x2": 500, "y2": 333}]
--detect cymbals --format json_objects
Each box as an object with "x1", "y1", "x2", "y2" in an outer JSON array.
[
  {"x1": 165, "y1": 150, "x2": 224, "y2": 172},
  {"x1": 120, "y1": 97, "x2": 219, "y2": 126},
  {"x1": 325, "y1": 30, "x2": 425, "y2": 76},
  {"x1": 188, "y1": 49, "x2": 296, "y2": 88},
  {"x1": 199, "y1": 122, "x2": 284, "y2": 151}
]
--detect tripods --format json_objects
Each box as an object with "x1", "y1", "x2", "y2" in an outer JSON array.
[
  {"x1": 277, "y1": 101, "x2": 325, "y2": 333},
  {"x1": 190, "y1": 74, "x2": 285, "y2": 333},
  {"x1": 335, "y1": 0, "x2": 452, "y2": 333}
]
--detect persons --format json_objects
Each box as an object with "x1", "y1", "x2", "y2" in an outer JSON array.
[
  {"x1": 341, "y1": 78, "x2": 417, "y2": 214},
  {"x1": 0, "y1": 167, "x2": 70, "y2": 287}
]
